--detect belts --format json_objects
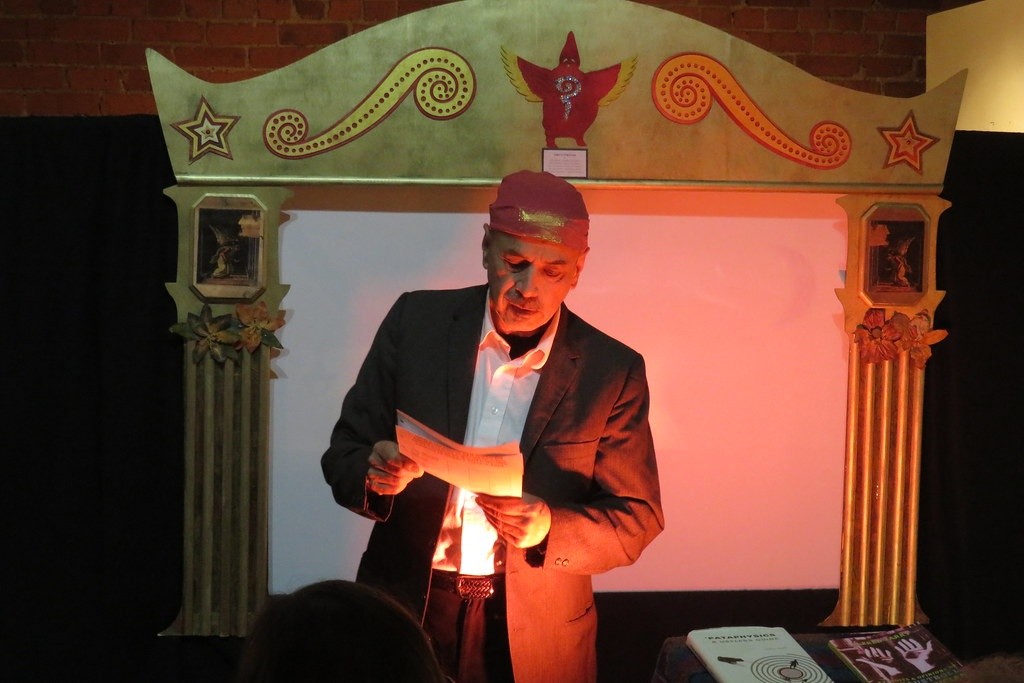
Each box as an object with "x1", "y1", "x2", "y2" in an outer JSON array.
[{"x1": 432, "y1": 569, "x2": 506, "y2": 600}]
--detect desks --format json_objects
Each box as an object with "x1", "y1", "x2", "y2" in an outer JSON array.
[{"x1": 647, "y1": 632, "x2": 862, "y2": 683}]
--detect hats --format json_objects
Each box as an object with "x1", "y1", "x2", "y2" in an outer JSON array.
[{"x1": 487, "y1": 169, "x2": 589, "y2": 249}]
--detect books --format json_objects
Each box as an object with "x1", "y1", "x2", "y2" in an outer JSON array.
[
  {"x1": 828, "y1": 621, "x2": 964, "y2": 683},
  {"x1": 686, "y1": 626, "x2": 835, "y2": 683}
]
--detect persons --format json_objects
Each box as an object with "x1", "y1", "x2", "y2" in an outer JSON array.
[
  {"x1": 238, "y1": 580, "x2": 445, "y2": 683},
  {"x1": 321, "y1": 170, "x2": 664, "y2": 683},
  {"x1": 955, "y1": 655, "x2": 1024, "y2": 683}
]
